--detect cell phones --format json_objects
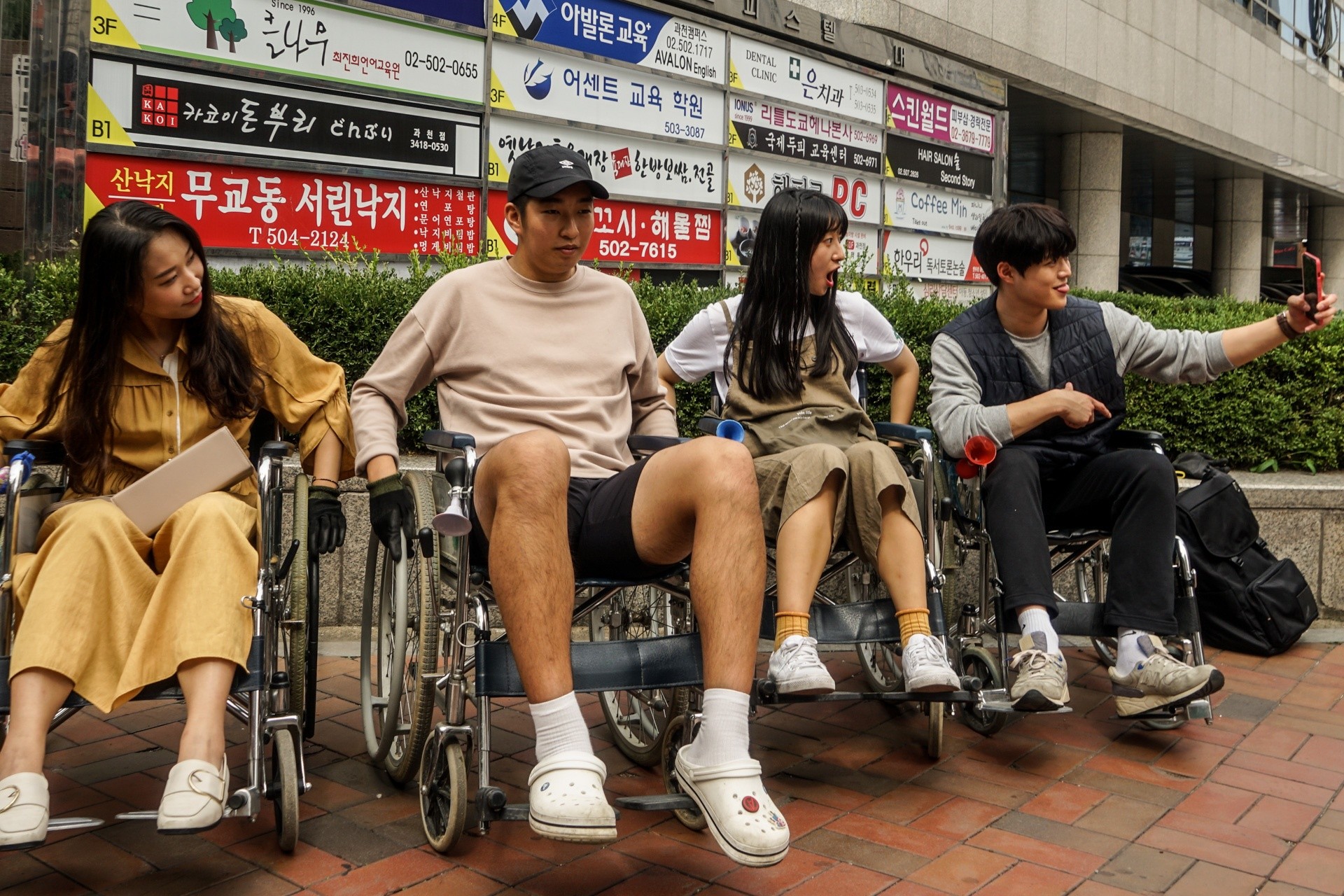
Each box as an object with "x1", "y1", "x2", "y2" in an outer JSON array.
[{"x1": 1301, "y1": 251, "x2": 1324, "y2": 326}]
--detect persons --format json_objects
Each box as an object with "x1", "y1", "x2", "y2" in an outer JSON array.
[
  {"x1": 350, "y1": 145, "x2": 792, "y2": 867},
  {"x1": 930, "y1": 201, "x2": 1336, "y2": 719},
  {"x1": 658, "y1": 189, "x2": 964, "y2": 694},
  {"x1": 0, "y1": 200, "x2": 359, "y2": 849}
]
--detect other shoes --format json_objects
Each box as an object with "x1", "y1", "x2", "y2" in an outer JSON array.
[
  {"x1": 156, "y1": 753, "x2": 230, "y2": 835},
  {"x1": 1, "y1": 772, "x2": 51, "y2": 851}
]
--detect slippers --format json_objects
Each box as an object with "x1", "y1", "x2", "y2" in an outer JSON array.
[
  {"x1": 674, "y1": 744, "x2": 790, "y2": 868},
  {"x1": 527, "y1": 753, "x2": 618, "y2": 844}
]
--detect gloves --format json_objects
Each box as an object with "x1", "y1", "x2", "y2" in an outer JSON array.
[
  {"x1": 1171, "y1": 451, "x2": 1230, "y2": 480},
  {"x1": 366, "y1": 472, "x2": 415, "y2": 560},
  {"x1": 308, "y1": 486, "x2": 346, "y2": 561}
]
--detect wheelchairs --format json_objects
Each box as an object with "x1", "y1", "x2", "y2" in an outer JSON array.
[
  {"x1": 0, "y1": 412, "x2": 321, "y2": 858},
  {"x1": 359, "y1": 361, "x2": 1212, "y2": 856}
]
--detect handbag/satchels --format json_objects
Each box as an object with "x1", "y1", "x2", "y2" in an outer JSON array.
[{"x1": 110, "y1": 426, "x2": 254, "y2": 538}]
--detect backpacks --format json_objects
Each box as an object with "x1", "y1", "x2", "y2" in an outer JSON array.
[{"x1": 1174, "y1": 467, "x2": 1319, "y2": 655}]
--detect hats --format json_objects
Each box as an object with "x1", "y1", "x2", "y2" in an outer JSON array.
[{"x1": 507, "y1": 145, "x2": 610, "y2": 202}]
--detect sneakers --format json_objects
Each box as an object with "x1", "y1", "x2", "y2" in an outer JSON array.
[
  {"x1": 767, "y1": 634, "x2": 835, "y2": 696},
  {"x1": 901, "y1": 633, "x2": 961, "y2": 693},
  {"x1": 1009, "y1": 628, "x2": 1070, "y2": 712},
  {"x1": 1108, "y1": 634, "x2": 1225, "y2": 718}
]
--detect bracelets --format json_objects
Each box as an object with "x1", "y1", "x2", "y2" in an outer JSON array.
[
  {"x1": 1277, "y1": 311, "x2": 1304, "y2": 340},
  {"x1": 311, "y1": 476, "x2": 340, "y2": 488}
]
